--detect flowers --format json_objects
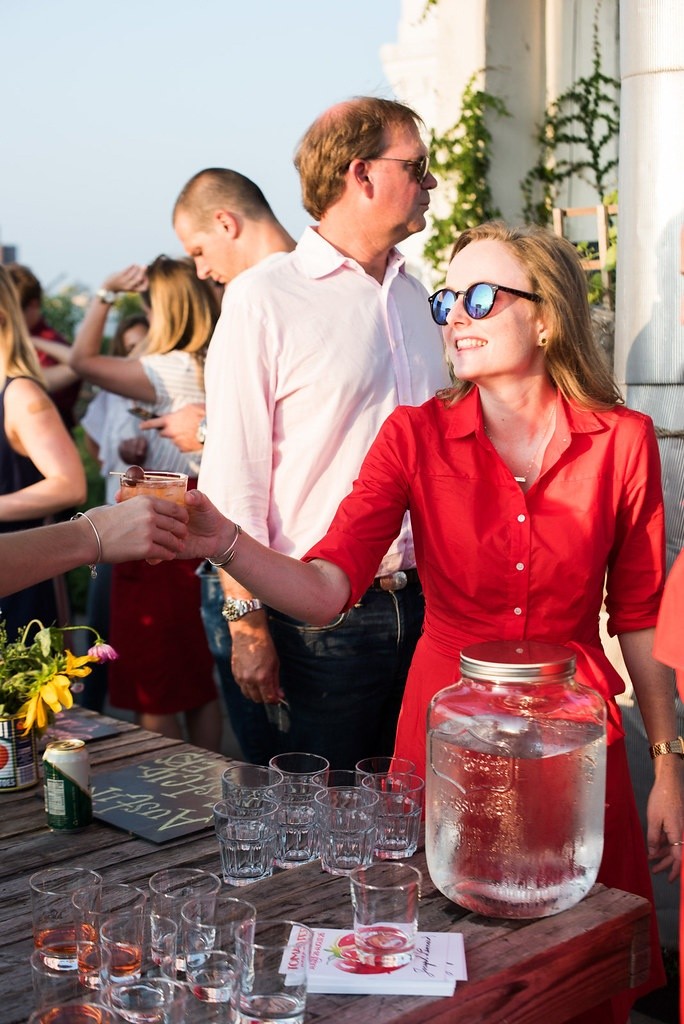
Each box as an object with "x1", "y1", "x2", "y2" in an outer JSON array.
[{"x1": 0, "y1": 620, "x2": 119, "y2": 737}]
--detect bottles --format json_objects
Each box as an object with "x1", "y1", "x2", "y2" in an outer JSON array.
[{"x1": 424, "y1": 640, "x2": 608, "y2": 919}]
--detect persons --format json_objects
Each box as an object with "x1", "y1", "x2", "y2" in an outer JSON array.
[
  {"x1": 10, "y1": 264, "x2": 82, "y2": 440},
  {"x1": 201, "y1": 95, "x2": 459, "y2": 789},
  {"x1": 0, "y1": 262, "x2": 88, "y2": 520},
  {"x1": 653, "y1": 545, "x2": 684, "y2": 703},
  {"x1": 69, "y1": 256, "x2": 228, "y2": 759},
  {"x1": 79, "y1": 315, "x2": 152, "y2": 506},
  {"x1": 0, "y1": 496, "x2": 189, "y2": 609},
  {"x1": 138, "y1": 166, "x2": 300, "y2": 452},
  {"x1": 119, "y1": 218, "x2": 684, "y2": 1024}
]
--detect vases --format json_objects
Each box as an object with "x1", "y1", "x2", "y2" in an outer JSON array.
[{"x1": 0, "y1": 712, "x2": 44, "y2": 792}]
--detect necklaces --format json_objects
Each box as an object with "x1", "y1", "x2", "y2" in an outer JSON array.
[{"x1": 478, "y1": 393, "x2": 557, "y2": 483}]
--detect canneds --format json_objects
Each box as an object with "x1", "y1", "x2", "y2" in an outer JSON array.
[{"x1": 41, "y1": 739, "x2": 92, "y2": 833}]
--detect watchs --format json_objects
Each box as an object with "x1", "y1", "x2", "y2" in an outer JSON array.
[
  {"x1": 651, "y1": 735, "x2": 684, "y2": 757},
  {"x1": 97, "y1": 290, "x2": 118, "y2": 306},
  {"x1": 197, "y1": 417, "x2": 209, "y2": 446},
  {"x1": 221, "y1": 597, "x2": 267, "y2": 619}
]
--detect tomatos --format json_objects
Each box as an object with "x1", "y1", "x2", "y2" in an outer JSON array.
[{"x1": 325, "y1": 925, "x2": 412, "y2": 974}]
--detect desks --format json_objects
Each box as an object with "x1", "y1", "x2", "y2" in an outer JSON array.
[{"x1": 0, "y1": 703, "x2": 666, "y2": 1024}]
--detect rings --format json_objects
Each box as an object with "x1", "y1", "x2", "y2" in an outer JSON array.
[{"x1": 670, "y1": 840, "x2": 684, "y2": 846}]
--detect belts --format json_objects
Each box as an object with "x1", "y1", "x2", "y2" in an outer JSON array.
[{"x1": 366, "y1": 568, "x2": 420, "y2": 598}]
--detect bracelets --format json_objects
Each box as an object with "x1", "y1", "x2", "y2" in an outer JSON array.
[
  {"x1": 69, "y1": 511, "x2": 104, "y2": 579},
  {"x1": 205, "y1": 523, "x2": 244, "y2": 569}
]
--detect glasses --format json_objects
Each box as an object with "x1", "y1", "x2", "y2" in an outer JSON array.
[
  {"x1": 372, "y1": 155, "x2": 431, "y2": 185},
  {"x1": 427, "y1": 280, "x2": 542, "y2": 327},
  {"x1": 147, "y1": 254, "x2": 171, "y2": 274}
]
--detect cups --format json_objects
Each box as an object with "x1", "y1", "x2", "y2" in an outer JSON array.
[
  {"x1": 119, "y1": 470, "x2": 188, "y2": 550},
  {"x1": 211, "y1": 752, "x2": 424, "y2": 887},
  {"x1": 26, "y1": 867, "x2": 313, "y2": 1024},
  {"x1": 348, "y1": 861, "x2": 423, "y2": 968}
]
